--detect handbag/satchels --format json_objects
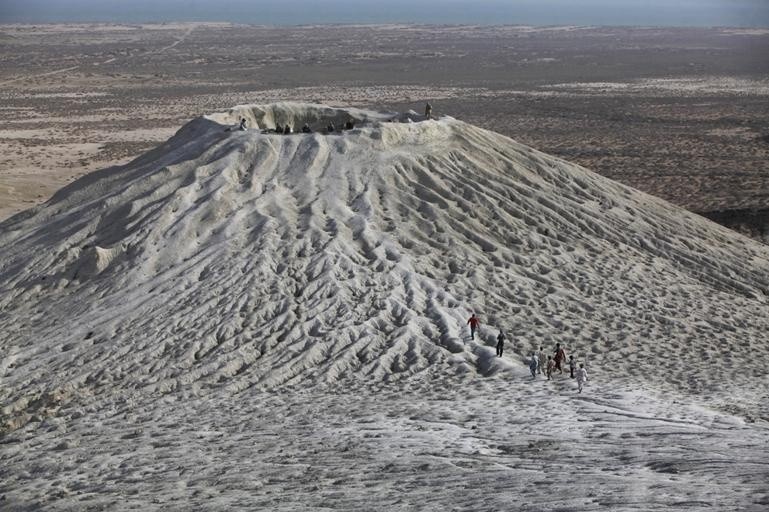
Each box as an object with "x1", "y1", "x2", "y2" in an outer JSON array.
[
  {"x1": 553, "y1": 357, "x2": 559, "y2": 362},
  {"x1": 547, "y1": 369, "x2": 550, "y2": 373}
]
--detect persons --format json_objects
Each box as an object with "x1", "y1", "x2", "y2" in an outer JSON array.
[
  {"x1": 574, "y1": 363, "x2": 589, "y2": 394},
  {"x1": 284, "y1": 124, "x2": 292, "y2": 134},
  {"x1": 345, "y1": 118, "x2": 354, "y2": 129},
  {"x1": 529, "y1": 350, "x2": 538, "y2": 380},
  {"x1": 546, "y1": 355, "x2": 556, "y2": 381},
  {"x1": 327, "y1": 121, "x2": 335, "y2": 133},
  {"x1": 274, "y1": 122, "x2": 284, "y2": 133},
  {"x1": 537, "y1": 345, "x2": 547, "y2": 376},
  {"x1": 566, "y1": 355, "x2": 577, "y2": 378},
  {"x1": 495, "y1": 327, "x2": 507, "y2": 359},
  {"x1": 424, "y1": 102, "x2": 432, "y2": 120},
  {"x1": 301, "y1": 122, "x2": 313, "y2": 133},
  {"x1": 467, "y1": 313, "x2": 481, "y2": 341},
  {"x1": 223, "y1": 116, "x2": 248, "y2": 132},
  {"x1": 553, "y1": 342, "x2": 566, "y2": 374}
]
